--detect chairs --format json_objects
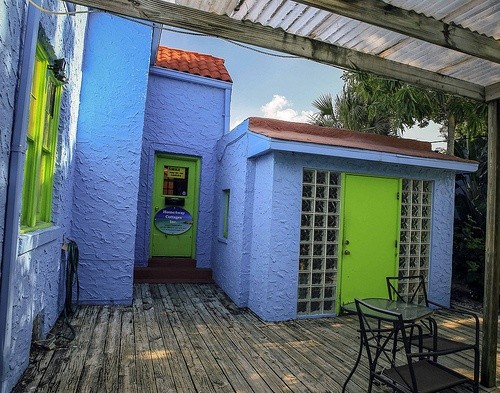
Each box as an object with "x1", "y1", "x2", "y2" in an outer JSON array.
[
  {"x1": 386, "y1": 275, "x2": 480, "y2": 363},
  {"x1": 354, "y1": 297, "x2": 480, "y2": 393}
]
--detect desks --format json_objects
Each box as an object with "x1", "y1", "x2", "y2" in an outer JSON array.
[{"x1": 340, "y1": 298, "x2": 434, "y2": 393}]
